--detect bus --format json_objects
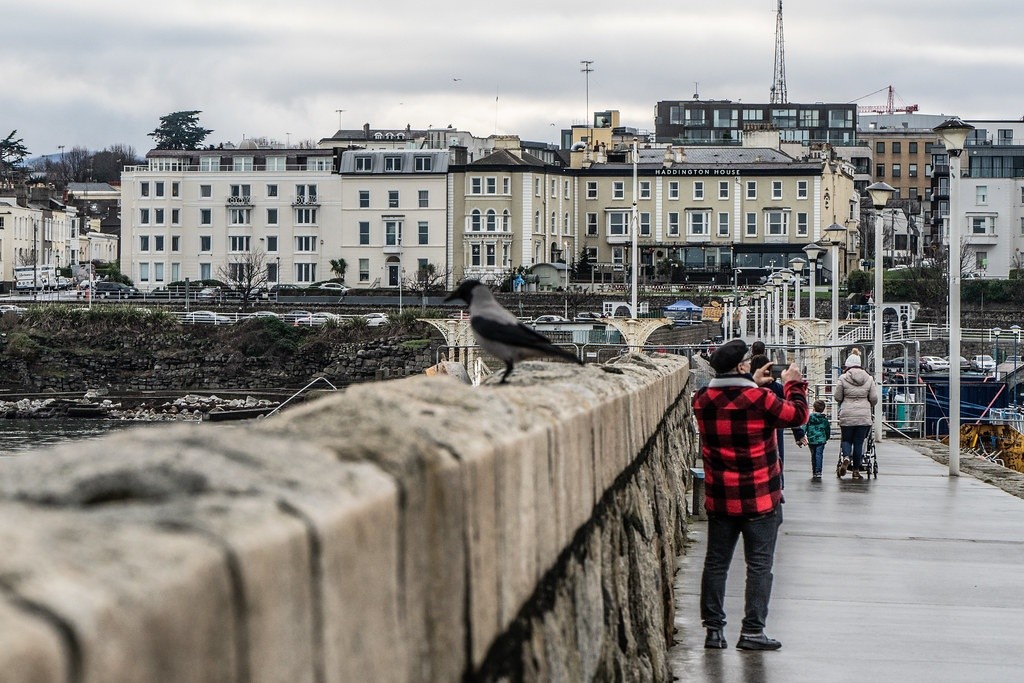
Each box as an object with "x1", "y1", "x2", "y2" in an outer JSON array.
[{"x1": 13, "y1": 263, "x2": 100, "y2": 298}]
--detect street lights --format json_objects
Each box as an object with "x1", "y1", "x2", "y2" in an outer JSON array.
[
  {"x1": 768, "y1": 272, "x2": 783, "y2": 342},
  {"x1": 931, "y1": 115, "x2": 976, "y2": 476},
  {"x1": 801, "y1": 242, "x2": 823, "y2": 317},
  {"x1": 751, "y1": 290, "x2": 761, "y2": 341},
  {"x1": 758, "y1": 287, "x2": 768, "y2": 342},
  {"x1": 824, "y1": 223, "x2": 848, "y2": 425},
  {"x1": 866, "y1": 177, "x2": 897, "y2": 444},
  {"x1": 741, "y1": 298, "x2": 749, "y2": 339},
  {"x1": 738, "y1": 298, "x2": 744, "y2": 339},
  {"x1": 778, "y1": 267, "x2": 795, "y2": 364},
  {"x1": 722, "y1": 294, "x2": 729, "y2": 345},
  {"x1": 727, "y1": 293, "x2": 736, "y2": 341},
  {"x1": 762, "y1": 282, "x2": 774, "y2": 361},
  {"x1": 1010, "y1": 324, "x2": 1022, "y2": 419},
  {"x1": 992, "y1": 327, "x2": 1002, "y2": 382},
  {"x1": 788, "y1": 256, "x2": 807, "y2": 369}
]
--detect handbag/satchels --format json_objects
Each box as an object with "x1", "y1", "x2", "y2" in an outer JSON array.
[{"x1": 851, "y1": 438, "x2": 868, "y2": 456}]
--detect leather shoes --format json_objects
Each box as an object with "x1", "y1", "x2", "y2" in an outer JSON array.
[
  {"x1": 705, "y1": 628, "x2": 727, "y2": 648},
  {"x1": 737, "y1": 632, "x2": 783, "y2": 650}
]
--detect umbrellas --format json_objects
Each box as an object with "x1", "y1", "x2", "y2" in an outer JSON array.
[{"x1": 883, "y1": 357, "x2": 916, "y2": 367}]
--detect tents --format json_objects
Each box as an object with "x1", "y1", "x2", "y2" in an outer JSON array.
[{"x1": 662, "y1": 299, "x2": 702, "y2": 320}]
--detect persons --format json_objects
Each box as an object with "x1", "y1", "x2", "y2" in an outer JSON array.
[
  {"x1": 833, "y1": 349, "x2": 879, "y2": 479},
  {"x1": 903, "y1": 320, "x2": 909, "y2": 339},
  {"x1": 885, "y1": 320, "x2": 892, "y2": 339},
  {"x1": 690, "y1": 339, "x2": 809, "y2": 650},
  {"x1": 752, "y1": 341, "x2": 765, "y2": 355},
  {"x1": 801, "y1": 400, "x2": 830, "y2": 478},
  {"x1": 75, "y1": 286, "x2": 89, "y2": 300},
  {"x1": 751, "y1": 355, "x2": 809, "y2": 502},
  {"x1": 895, "y1": 367, "x2": 913, "y2": 372},
  {"x1": 887, "y1": 368, "x2": 892, "y2": 372},
  {"x1": 920, "y1": 364, "x2": 933, "y2": 372}
]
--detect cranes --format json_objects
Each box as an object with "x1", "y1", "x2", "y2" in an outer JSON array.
[{"x1": 846, "y1": 85, "x2": 919, "y2": 115}]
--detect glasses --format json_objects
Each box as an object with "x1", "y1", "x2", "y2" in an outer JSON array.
[{"x1": 737, "y1": 354, "x2": 752, "y2": 369}]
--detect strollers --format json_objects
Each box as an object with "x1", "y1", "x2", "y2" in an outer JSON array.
[{"x1": 836, "y1": 405, "x2": 878, "y2": 480}]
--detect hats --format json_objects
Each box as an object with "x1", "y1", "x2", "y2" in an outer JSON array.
[
  {"x1": 845, "y1": 348, "x2": 861, "y2": 367},
  {"x1": 710, "y1": 339, "x2": 749, "y2": 374}
]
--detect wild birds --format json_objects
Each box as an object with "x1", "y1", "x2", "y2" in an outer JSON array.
[
  {"x1": 454, "y1": 78, "x2": 461, "y2": 81},
  {"x1": 550, "y1": 123, "x2": 554, "y2": 126},
  {"x1": 443, "y1": 280, "x2": 584, "y2": 383}
]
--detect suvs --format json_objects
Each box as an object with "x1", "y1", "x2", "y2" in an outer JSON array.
[{"x1": 95, "y1": 281, "x2": 140, "y2": 299}]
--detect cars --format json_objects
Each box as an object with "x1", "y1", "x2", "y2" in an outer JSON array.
[
  {"x1": 882, "y1": 355, "x2": 931, "y2": 375},
  {"x1": 240, "y1": 310, "x2": 346, "y2": 329},
  {"x1": 198, "y1": 283, "x2": 248, "y2": 304},
  {"x1": 319, "y1": 282, "x2": 352, "y2": 296},
  {"x1": 535, "y1": 314, "x2": 571, "y2": 333},
  {"x1": 943, "y1": 355, "x2": 971, "y2": 372},
  {"x1": 269, "y1": 284, "x2": 306, "y2": 301},
  {"x1": 185, "y1": 310, "x2": 231, "y2": 328},
  {"x1": 576, "y1": 311, "x2": 602, "y2": 324},
  {"x1": 970, "y1": 354, "x2": 997, "y2": 374},
  {"x1": 360, "y1": 312, "x2": 390, "y2": 329},
  {"x1": 0, "y1": 305, "x2": 29, "y2": 317},
  {"x1": 248, "y1": 287, "x2": 270, "y2": 301},
  {"x1": 887, "y1": 260, "x2": 981, "y2": 281},
  {"x1": 922, "y1": 356, "x2": 949, "y2": 373}
]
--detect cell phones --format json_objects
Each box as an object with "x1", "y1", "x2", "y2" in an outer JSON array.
[{"x1": 769, "y1": 364, "x2": 790, "y2": 378}]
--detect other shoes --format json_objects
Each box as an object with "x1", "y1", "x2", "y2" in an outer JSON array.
[
  {"x1": 839, "y1": 457, "x2": 850, "y2": 475},
  {"x1": 853, "y1": 472, "x2": 863, "y2": 478},
  {"x1": 813, "y1": 474, "x2": 817, "y2": 478},
  {"x1": 816, "y1": 472, "x2": 821, "y2": 478}
]
